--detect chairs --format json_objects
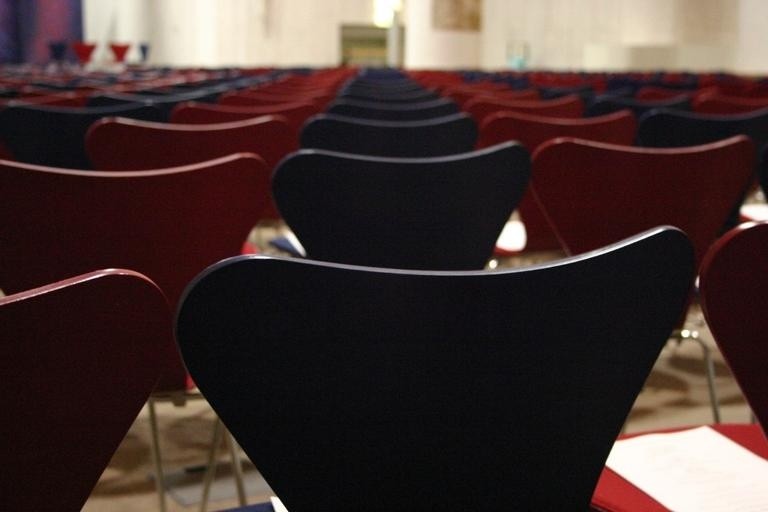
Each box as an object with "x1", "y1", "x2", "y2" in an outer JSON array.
[
  {"x1": 2, "y1": 147, "x2": 269, "y2": 512},
  {"x1": 271, "y1": 139, "x2": 536, "y2": 278},
  {"x1": 2, "y1": 36, "x2": 768, "y2": 243},
  {"x1": 175, "y1": 222, "x2": 701, "y2": 511},
  {"x1": 4, "y1": 259, "x2": 173, "y2": 509},
  {"x1": 579, "y1": 219, "x2": 767, "y2": 507},
  {"x1": 529, "y1": 133, "x2": 757, "y2": 270}
]
